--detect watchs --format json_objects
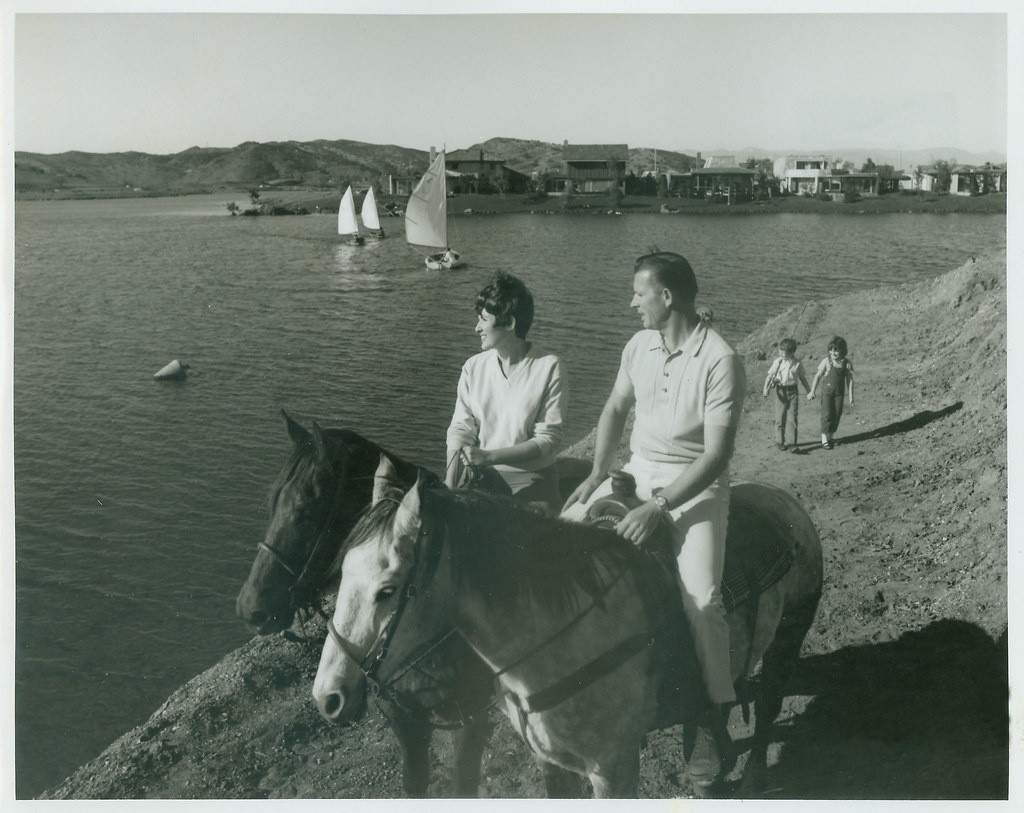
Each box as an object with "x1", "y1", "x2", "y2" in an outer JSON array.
[{"x1": 654, "y1": 493, "x2": 672, "y2": 514}]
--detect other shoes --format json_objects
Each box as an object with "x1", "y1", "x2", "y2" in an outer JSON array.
[
  {"x1": 789, "y1": 445, "x2": 799, "y2": 453},
  {"x1": 829, "y1": 440, "x2": 834, "y2": 446},
  {"x1": 777, "y1": 443, "x2": 787, "y2": 451},
  {"x1": 821, "y1": 442, "x2": 830, "y2": 449}
]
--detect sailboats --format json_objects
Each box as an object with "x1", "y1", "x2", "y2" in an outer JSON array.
[
  {"x1": 401, "y1": 143, "x2": 467, "y2": 271},
  {"x1": 361, "y1": 185, "x2": 385, "y2": 241},
  {"x1": 336, "y1": 183, "x2": 366, "y2": 247}
]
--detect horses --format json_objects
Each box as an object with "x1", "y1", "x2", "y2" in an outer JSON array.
[{"x1": 234, "y1": 409, "x2": 824, "y2": 800}]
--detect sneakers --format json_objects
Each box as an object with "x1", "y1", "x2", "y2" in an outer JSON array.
[{"x1": 687, "y1": 715, "x2": 722, "y2": 781}]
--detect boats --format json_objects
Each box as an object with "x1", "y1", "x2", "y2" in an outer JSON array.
[{"x1": 660, "y1": 206, "x2": 680, "y2": 214}]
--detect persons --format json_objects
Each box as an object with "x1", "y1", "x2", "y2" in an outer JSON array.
[
  {"x1": 558, "y1": 252, "x2": 748, "y2": 786},
  {"x1": 444, "y1": 247, "x2": 456, "y2": 263},
  {"x1": 763, "y1": 339, "x2": 816, "y2": 451},
  {"x1": 807, "y1": 336, "x2": 855, "y2": 451},
  {"x1": 444, "y1": 267, "x2": 568, "y2": 494}
]
